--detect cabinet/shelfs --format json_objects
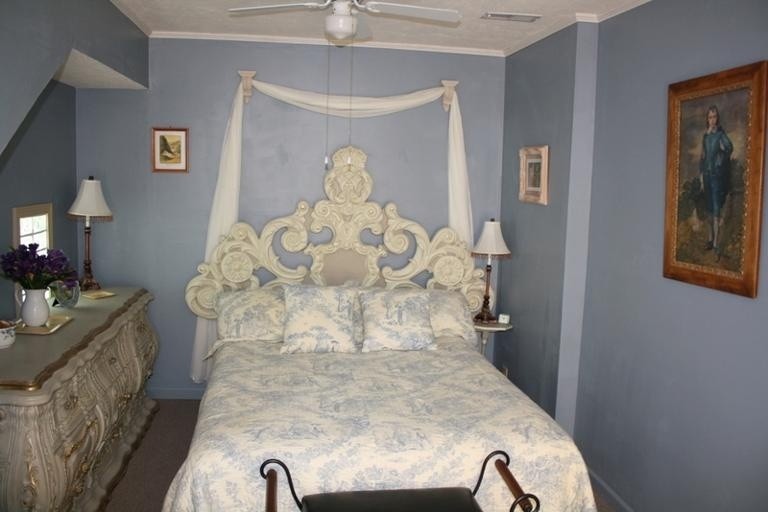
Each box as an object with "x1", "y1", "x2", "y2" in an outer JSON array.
[{"x1": 1, "y1": 282, "x2": 162, "y2": 512}]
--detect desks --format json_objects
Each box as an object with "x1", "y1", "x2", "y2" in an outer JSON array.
[{"x1": 471, "y1": 317, "x2": 514, "y2": 358}]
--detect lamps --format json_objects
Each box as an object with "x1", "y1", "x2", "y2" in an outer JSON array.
[
  {"x1": 468, "y1": 216, "x2": 513, "y2": 325},
  {"x1": 322, "y1": 0, "x2": 362, "y2": 48},
  {"x1": 63, "y1": 173, "x2": 116, "y2": 292}
]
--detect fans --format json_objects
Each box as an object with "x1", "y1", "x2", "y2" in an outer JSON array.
[{"x1": 222, "y1": 2, "x2": 467, "y2": 29}]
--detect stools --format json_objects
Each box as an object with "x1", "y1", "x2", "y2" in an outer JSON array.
[{"x1": 259, "y1": 446, "x2": 547, "y2": 510}]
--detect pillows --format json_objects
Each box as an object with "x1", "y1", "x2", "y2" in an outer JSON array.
[
  {"x1": 354, "y1": 285, "x2": 441, "y2": 353},
  {"x1": 208, "y1": 282, "x2": 284, "y2": 344},
  {"x1": 424, "y1": 283, "x2": 481, "y2": 344},
  {"x1": 277, "y1": 283, "x2": 360, "y2": 356}
]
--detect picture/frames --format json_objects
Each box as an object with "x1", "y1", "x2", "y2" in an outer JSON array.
[
  {"x1": 662, "y1": 59, "x2": 766, "y2": 303},
  {"x1": 514, "y1": 142, "x2": 551, "y2": 208},
  {"x1": 149, "y1": 125, "x2": 192, "y2": 175}
]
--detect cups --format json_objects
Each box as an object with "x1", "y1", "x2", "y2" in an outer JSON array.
[
  {"x1": 15, "y1": 286, "x2": 50, "y2": 327},
  {"x1": 0, "y1": 321, "x2": 17, "y2": 349}
]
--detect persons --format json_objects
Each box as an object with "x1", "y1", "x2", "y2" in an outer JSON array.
[{"x1": 699, "y1": 105, "x2": 734, "y2": 256}]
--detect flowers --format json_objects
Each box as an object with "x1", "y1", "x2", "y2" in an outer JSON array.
[{"x1": 2, "y1": 234, "x2": 76, "y2": 290}]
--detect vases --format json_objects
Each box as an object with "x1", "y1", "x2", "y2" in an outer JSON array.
[{"x1": 17, "y1": 289, "x2": 54, "y2": 328}]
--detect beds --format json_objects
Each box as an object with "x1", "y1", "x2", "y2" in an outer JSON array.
[{"x1": 159, "y1": 144, "x2": 599, "y2": 512}]
